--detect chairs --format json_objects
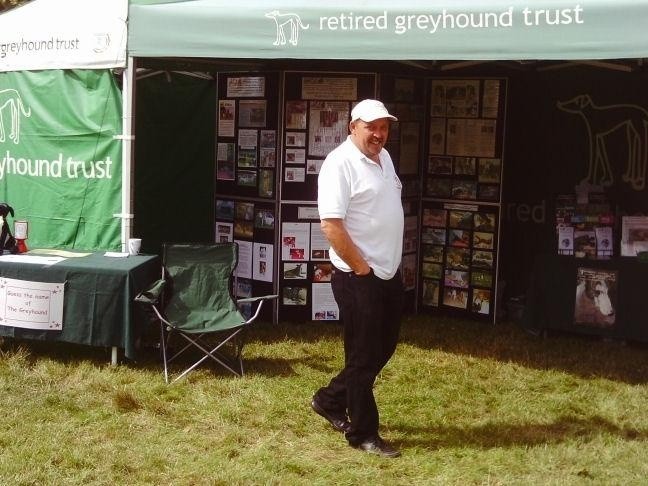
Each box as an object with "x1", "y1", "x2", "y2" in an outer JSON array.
[{"x1": 137, "y1": 242, "x2": 278, "y2": 387}]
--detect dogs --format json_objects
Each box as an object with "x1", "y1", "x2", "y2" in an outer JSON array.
[{"x1": 284, "y1": 264, "x2": 306, "y2": 278}]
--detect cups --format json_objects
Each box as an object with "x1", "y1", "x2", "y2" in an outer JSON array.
[{"x1": 128, "y1": 239, "x2": 142, "y2": 256}]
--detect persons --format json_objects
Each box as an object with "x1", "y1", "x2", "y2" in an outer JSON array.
[{"x1": 311, "y1": 98, "x2": 404, "y2": 458}]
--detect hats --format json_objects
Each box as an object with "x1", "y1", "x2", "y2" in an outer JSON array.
[{"x1": 351, "y1": 99, "x2": 398, "y2": 123}]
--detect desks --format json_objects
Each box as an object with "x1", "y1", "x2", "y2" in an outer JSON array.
[
  {"x1": 518, "y1": 251, "x2": 647, "y2": 337},
  {"x1": 0, "y1": 248, "x2": 159, "y2": 366}
]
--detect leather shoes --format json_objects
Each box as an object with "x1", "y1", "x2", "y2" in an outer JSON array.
[{"x1": 311, "y1": 400, "x2": 401, "y2": 458}]
